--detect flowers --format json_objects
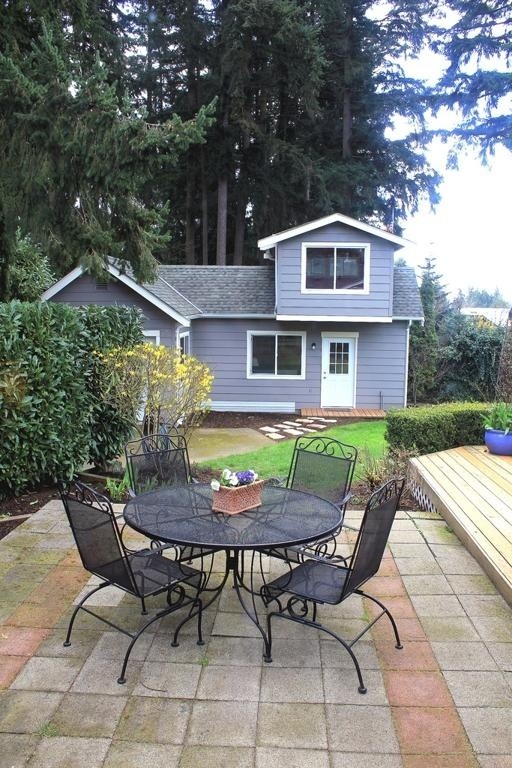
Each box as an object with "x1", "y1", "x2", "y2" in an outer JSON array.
[{"x1": 209, "y1": 468, "x2": 258, "y2": 491}]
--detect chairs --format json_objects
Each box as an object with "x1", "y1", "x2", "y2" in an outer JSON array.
[
  {"x1": 260, "y1": 476, "x2": 407, "y2": 693},
  {"x1": 124, "y1": 432, "x2": 201, "y2": 569},
  {"x1": 56, "y1": 477, "x2": 206, "y2": 682},
  {"x1": 233, "y1": 435, "x2": 358, "y2": 622}
]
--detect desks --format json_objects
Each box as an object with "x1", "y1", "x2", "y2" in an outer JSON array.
[{"x1": 123, "y1": 481, "x2": 342, "y2": 663}]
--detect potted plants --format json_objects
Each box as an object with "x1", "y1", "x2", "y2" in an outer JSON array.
[{"x1": 479, "y1": 398, "x2": 512, "y2": 455}]
